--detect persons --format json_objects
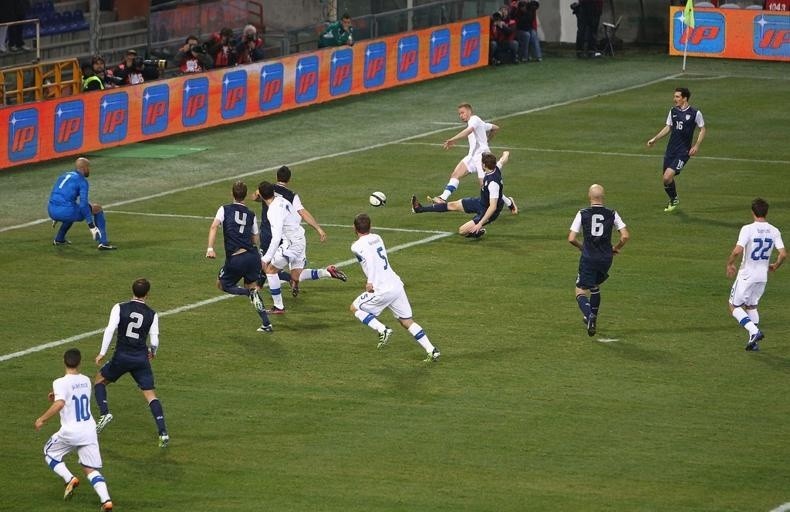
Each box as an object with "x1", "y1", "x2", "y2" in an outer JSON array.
[
  {"x1": 113, "y1": 50, "x2": 158, "y2": 84},
  {"x1": 317, "y1": 14, "x2": 353, "y2": 51},
  {"x1": 47, "y1": 158, "x2": 116, "y2": 250},
  {"x1": 350, "y1": 214, "x2": 441, "y2": 364},
  {"x1": 92, "y1": 279, "x2": 169, "y2": 447},
  {"x1": 202, "y1": 28, "x2": 241, "y2": 70},
  {"x1": 235, "y1": 24, "x2": 263, "y2": 64},
  {"x1": 426, "y1": 103, "x2": 499, "y2": 204},
  {"x1": 34, "y1": 348, "x2": 113, "y2": 512},
  {"x1": 489, "y1": 1, "x2": 543, "y2": 69},
  {"x1": 565, "y1": 182, "x2": 628, "y2": 337},
  {"x1": 83, "y1": 56, "x2": 119, "y2": 89},
  {"x1": 251, "y1": 164, "x2": 326, "y2": 291},
  {"x1": 571, "y1": 0, "x2": 604, "y2": 60},
  {"x1": 645, "y1": 87, "x2": 706, "y2": 212},
  {"x1": 205, "y1": 182, "x2": 272, "y2": 332},
  {"x1": 174, "y1": 36, "x2": 212, "y2": 75},
  {"x1": 256, "y1": 181, "x2": 347, "y2": 315},
  {"x1": 726, "y1": 196, "x2": 785, "y2": 353},
  {"x1": 409, "y1": 149, "x2": 511, "y2": 237},
  {"x1": 5, "y1": 1, "x2": 32, "y2": 53}
]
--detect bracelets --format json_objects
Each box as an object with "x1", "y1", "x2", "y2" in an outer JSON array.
[{"x1": 207, "y1": 247, "x2": 214, "y2": 252}]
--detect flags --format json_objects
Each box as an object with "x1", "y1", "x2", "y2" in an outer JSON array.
[{"x1": 681, "y1": 1, "x2": 695, "y2": 30}]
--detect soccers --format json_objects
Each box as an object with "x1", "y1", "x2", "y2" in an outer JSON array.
[{"x1": 368, "y1": 190, "x2": 387, "y2": 206}]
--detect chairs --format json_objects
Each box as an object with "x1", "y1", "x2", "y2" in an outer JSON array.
[
  {"x1": 0, "y1": 0, "x2": 93, "y2": 41},
  {"x1": 684, "y1": 1, "x2": 765, "y2": 13}
]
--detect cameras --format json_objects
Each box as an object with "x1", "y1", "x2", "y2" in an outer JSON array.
[
  {"x1": 247, "y1": 33, "x2": 253, "y2": 41},
  {"x1": 224, "y1": 37, "x2": 237, "y2": 46}
]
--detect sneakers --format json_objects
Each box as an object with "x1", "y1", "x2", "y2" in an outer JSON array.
[
  {"x1": 92, "y1": 413, "x2": 115, "y2": 435},
  {"x1": 581, "y1": 311, "x2": 600, "y2": 339},
  {"x1": 579, "y1": 49, "x2": 601, "y2": 61},
  {"x1": 324, "y1": 265, "x2": 345, "y2": 281},
  {"x1": 490, "y1": 56, "x2": 545, "y2": 65},
  {"x1": 156, "y1": 431, "x2": 173, "y2": 451},
  {"x1": 421, "y1": 348, "x2": 443, "y2": 362},
  {"x1": 98, "y1": 499, "x2": 115, "y2": 512},
  {"x1": 52, "y1": 238, "x2": 70, "y2": 245},
  {"x1": 471, "y1": 228, "x2": 486, "y2": 238},
  {"x1": 372, "y1": 327, "x2": 394, "y2": 351},
  {"x1": 662, "y1": 195, "x2": 679, "y2": 212},
  {"x1": 424, "y1": 195, "x2": 444, "y2": 206},
  {"x1": 411, "y1": 195, "x2": 422, "y2": 215},
  {"x1": 95, "y1": 243, "x2": 117, "y2": 250},
  {"x1": 745, "y1": 326, "x2": 762, "y2": 353},
  {"x1": 250, "y1": 282, "x2": 300, "y2": 335},
  {"x1": 508, "y1": 196, "x2": 518, "y2": 214},
  {"x1": 60, "y1": 475, "x2": 79, "y2": 502}
]
